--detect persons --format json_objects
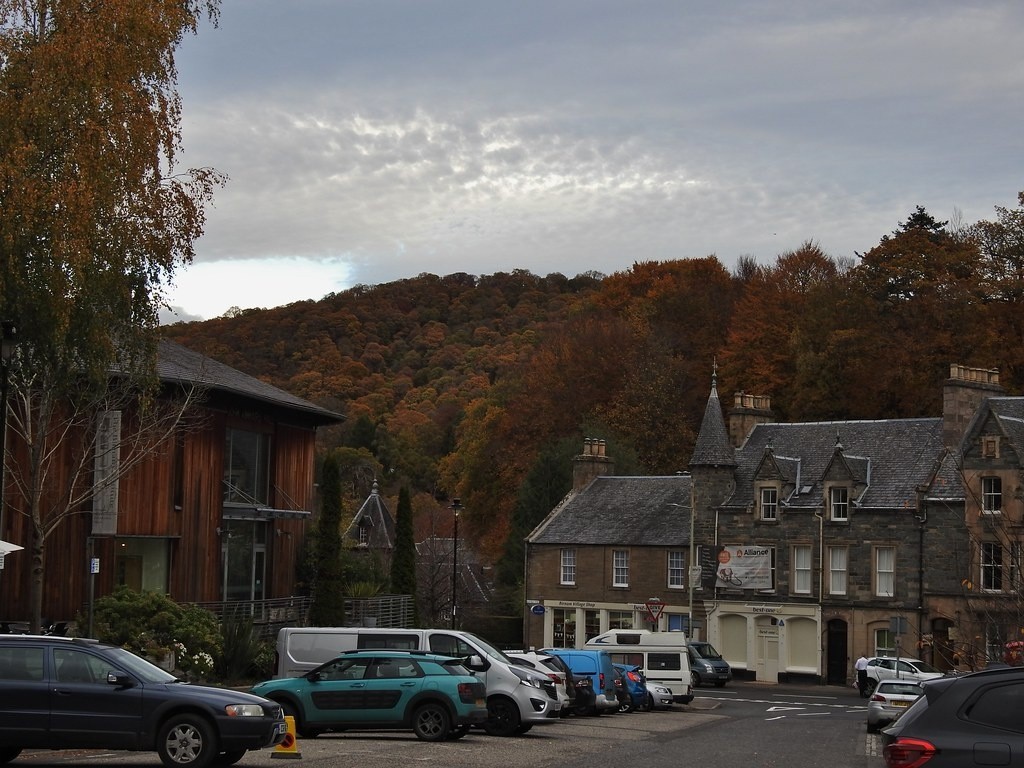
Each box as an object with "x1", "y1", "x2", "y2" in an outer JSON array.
[{"x1": 855, "y1": 651, "x2": 868, "y2": 698}]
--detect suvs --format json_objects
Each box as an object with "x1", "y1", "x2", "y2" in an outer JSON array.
[
  {"x1": 855, "y1": 656, "x2": 946, "y2": 697},
  {"x1": 0, "y1": 634, "x2": 288, "y2": 768},
  {"x1": 248, "y1": 648, "x2": 488, "y2": 743},
  {"x1": 880, "y1": 666, "x2": 1024, "y2": 767}
]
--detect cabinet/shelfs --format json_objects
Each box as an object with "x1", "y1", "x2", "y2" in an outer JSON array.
[
  {"x1": 586, "y1": 624, "x2": 600, "y2": 634},
  {"x1": 555, "y1": 623, "x2": 575, "y2": 641}
]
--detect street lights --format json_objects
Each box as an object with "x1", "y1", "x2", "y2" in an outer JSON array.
[
  {"x1": 666, "y1": 481, "x2": 695, "y2": 638},
  {"x1": 447, "y1": 498, "x2": 465, "y2": 630}
]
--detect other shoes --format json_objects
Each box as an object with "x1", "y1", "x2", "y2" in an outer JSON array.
[{"x1": 860, "y1": 693, "x2": 863, "y2": 697}]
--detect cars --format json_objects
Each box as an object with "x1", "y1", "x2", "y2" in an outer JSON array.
[
  {"x1": 467, "y1": 648, "x2": 675, "y2": 718},
  {"x1": 867, "y1": 679, "x2": 924, "y2": 733}
]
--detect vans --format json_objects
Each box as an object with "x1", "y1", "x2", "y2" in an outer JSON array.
[
  {"x1": 271, "y1": 627, "x2": 561, "y2": 737},
  {"x1": 581, "y1": 628, "x2": 734, "y2": 704}
]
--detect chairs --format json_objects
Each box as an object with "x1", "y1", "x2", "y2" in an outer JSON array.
[
  {"x1": 379, "y1": 664, "x2": 395, "y2": 677},
  {"x1": 58, "y1": 656, "x2": 85, "y2": 683}
]
